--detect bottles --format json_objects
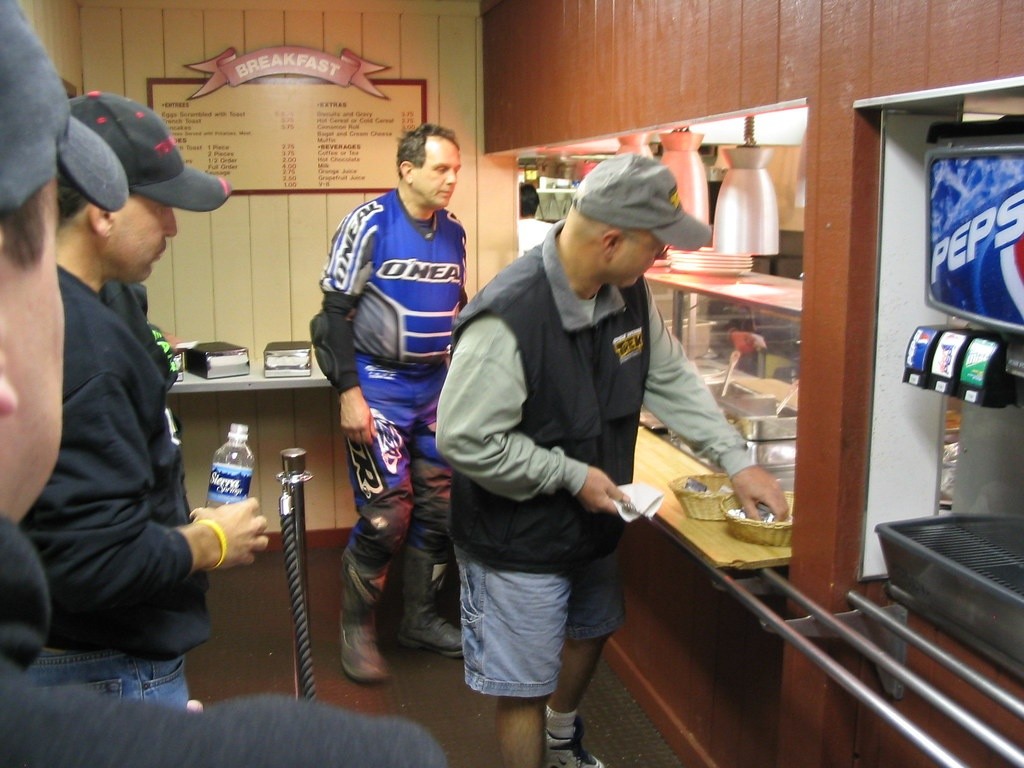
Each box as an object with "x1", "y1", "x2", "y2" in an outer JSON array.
[{"x1": 204, "y1": 423, "x2": 255, "y2": 509}]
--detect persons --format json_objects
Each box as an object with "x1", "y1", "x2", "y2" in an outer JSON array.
[
  {"x1": 0, "y1": 1, "x2": 449, "y2": 768},
  {"x1": 19, "y1": 88, "x2": 268, "y2": 714},
  {"x1": 308, "y1": 126, "x2": 468, "y2": 680},
  {"x1": 436, "y1": 153, "x2": 790, "y2": 768},
  {"x1": 148, "y1": 323, "x2": 184, "y2": 393}
]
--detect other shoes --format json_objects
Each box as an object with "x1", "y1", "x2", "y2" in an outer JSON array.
[{"x1": 545, "y1": 715, "x2": 605, "y2": 768}]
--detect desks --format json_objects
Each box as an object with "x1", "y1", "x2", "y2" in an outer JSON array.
[{"x1": 167, "y1": 361, "x2": 334, "y2": 393}]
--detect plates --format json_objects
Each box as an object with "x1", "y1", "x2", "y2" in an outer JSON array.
[{"x1": 653, "y1": 250, "x2": 754, "y2": 277}]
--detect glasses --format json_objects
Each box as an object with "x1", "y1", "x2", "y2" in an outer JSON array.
[{"x1": 602, "y1": 228, "x2": 670, "y2": 260}]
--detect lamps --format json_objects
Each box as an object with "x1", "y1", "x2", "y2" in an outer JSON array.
[{"x1": 617, "y1": 114, "x2": 780, "y2": 258}]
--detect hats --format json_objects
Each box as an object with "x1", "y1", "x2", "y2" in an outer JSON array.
[
  {"x1": 572, "y1": 152, "x2": 712, "y2": 251},
  {"x1": 0, "y1": 0, "x2": 128, "y2": 212},
  {"x1": 69, "y1": 90, "x2": 232, "y2": 212}
]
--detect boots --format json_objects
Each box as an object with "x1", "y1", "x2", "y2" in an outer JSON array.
[
  {"x1": 396, "y1": 544, "x2": 462, "y2": 657},
  {"x1": 338, "y1": 546, "x2": 393, "y2": 684}
]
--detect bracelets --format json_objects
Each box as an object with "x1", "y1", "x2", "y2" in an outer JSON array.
[{"x1": 197, "y1": 520, "x2": 227, "y2": 571}]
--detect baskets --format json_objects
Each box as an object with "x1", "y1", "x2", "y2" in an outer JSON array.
[
  {"x1": 670, "y1": 473, "x2": 733, "y2": 521},
  {"x1": 719, "y1": 491, "x2": 794, "y2": 546}
]
